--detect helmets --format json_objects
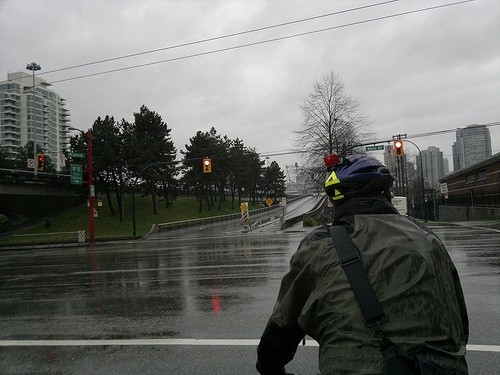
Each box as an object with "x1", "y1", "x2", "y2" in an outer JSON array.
[{"x1": 324, "y1": 156, "x2": 393, "y2": 205}]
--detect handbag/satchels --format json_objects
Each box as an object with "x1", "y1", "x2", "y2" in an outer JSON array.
[{"x1": 389, "y1": 354, "x2": 453, "y2": 375}]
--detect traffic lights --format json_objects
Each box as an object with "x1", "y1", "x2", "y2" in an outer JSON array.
[
  {"x1": 203, "y1": 158, "x2": 211, "y2": 174},
  {"x1": 394, "y1": 139, "x2": 403, "y2": 156},
  {"x1": 36, "y1": 154, "x2": 44, "y2": 171}
]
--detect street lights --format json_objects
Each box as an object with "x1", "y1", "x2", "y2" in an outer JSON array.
[
  {"x1": 68, "y1": 127, "x2": 94, "y2": 244},
  {"x1": 26, "y1": 61, "x2": 41, "y2": 171}
]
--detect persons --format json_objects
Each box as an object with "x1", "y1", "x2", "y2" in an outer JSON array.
[{"x1": 256, "y1": 154, "x2": 470, "y2": 375}]
